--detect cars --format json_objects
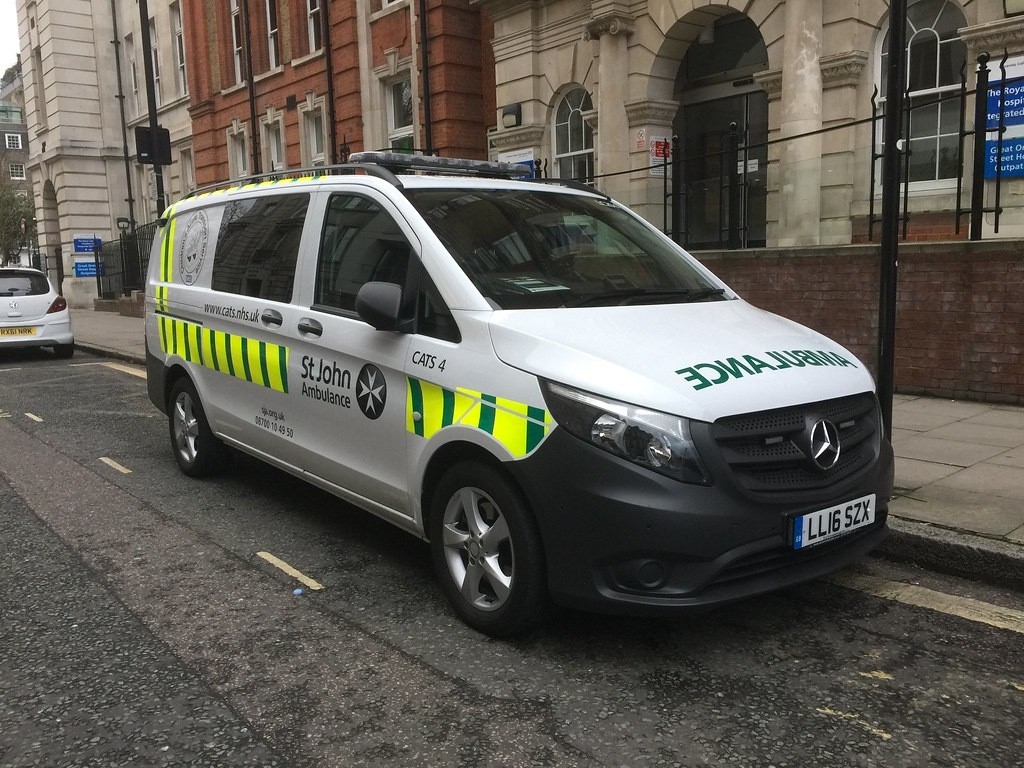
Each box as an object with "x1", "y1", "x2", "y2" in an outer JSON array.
[{"x1": 0, "y1": 268, "x2": 75, "y2": 359}]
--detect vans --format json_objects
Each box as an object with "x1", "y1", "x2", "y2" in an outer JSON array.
[{"x1": 144, "y1": 150, "x2": 895, "y2": 644}]
